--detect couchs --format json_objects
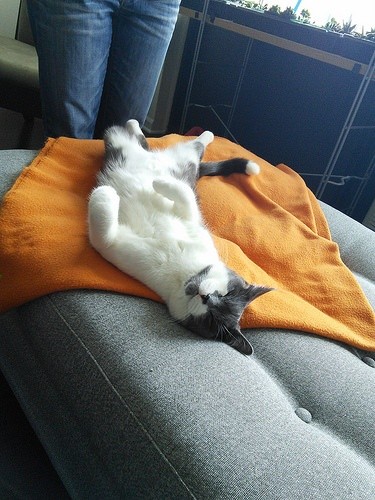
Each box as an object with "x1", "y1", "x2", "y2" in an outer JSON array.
[{"x1": 1, "y1": 131, "x2": 375, "y2": 500}]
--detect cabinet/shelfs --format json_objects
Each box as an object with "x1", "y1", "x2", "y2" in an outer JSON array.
[{"x1": 177, "y1": 0, "x2": 374, "y2": 227}]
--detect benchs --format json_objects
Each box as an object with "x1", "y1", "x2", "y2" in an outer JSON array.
[{"x1": 0, "y1": 36, "x2": 47, "y2": 149}]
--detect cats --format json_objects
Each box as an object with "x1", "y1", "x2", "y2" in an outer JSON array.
[{"x1": 87, "y1": 119, "x2": 275, "y2": 357}]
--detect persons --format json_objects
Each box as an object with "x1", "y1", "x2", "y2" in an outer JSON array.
[{"x1": 24, "y1": 0, "x2": 183, "y2": 139}]
